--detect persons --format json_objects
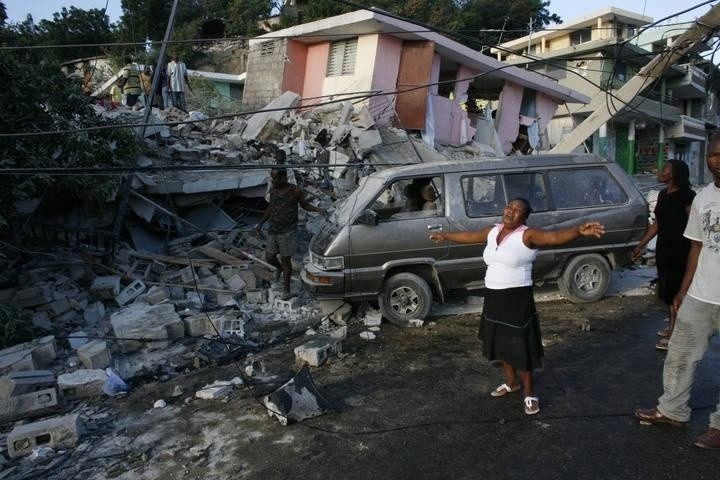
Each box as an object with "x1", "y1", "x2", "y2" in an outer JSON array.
[
  {"x1": 78, "y1": 48, "x2": 194, "y2": 112},
  {"x1": 633, "y1": 126, "x2": 719, "y2": 452},
  {"x1": 427, "y1": 194, "x2": 608, "y2": 418},
  {"x1": 264, "y1": 149, "x2": 298, "y2": 204},
  {"x1": 630, "y1": 156, "x2": 697, "y2": 352},
  {"x1": 250, "y1": 166, "x2": 328, "y2": 302}
]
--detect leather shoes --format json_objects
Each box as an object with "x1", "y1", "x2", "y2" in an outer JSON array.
[
  {"x1": 636, "y1": 409, "x2": 688, "y2": 426},
  {"x1": 693, "y1": 428, "x2": 720, "y2": 449}
]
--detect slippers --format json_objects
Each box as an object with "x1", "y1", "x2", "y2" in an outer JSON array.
[
  {"x1": 524, "y1": 395, "x2": 540, "y2": 415},
  {"x1": 491, "y1": 383, "x2": 521, "y2": 397},
  {"x1": 656, "y1": 337, "x2": 668, "y2": 349},
  {"x1": 658, "y1": 328, "x2": 670, "y2": 336}
]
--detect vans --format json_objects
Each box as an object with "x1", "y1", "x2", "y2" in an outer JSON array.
[{"x1": 300, "y1": 153, "x2": 649, "y2": 327}]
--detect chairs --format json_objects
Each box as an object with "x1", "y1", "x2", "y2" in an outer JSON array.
[
  {"x1": 398, "y1": 186, "x2": 415, "y2": 212},
  {"x1": 418, "y1": 187, "x2": 437, "y2": 211}
]
